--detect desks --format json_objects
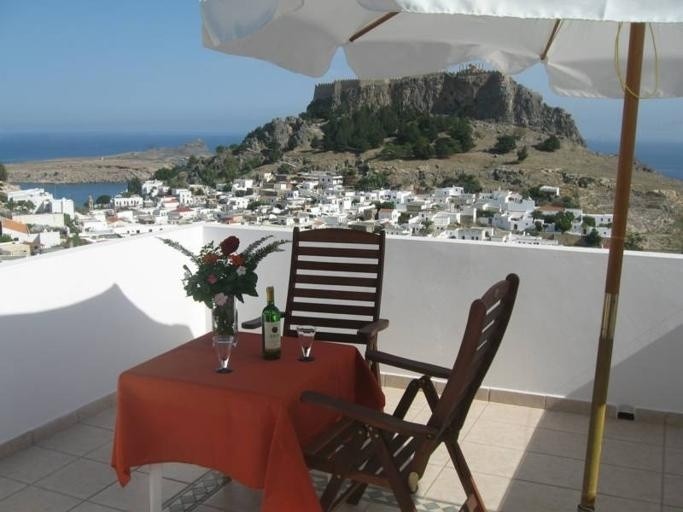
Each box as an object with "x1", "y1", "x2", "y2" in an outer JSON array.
[{"x1": 109, "y1": 330, "x2": 389, "y2": 511}]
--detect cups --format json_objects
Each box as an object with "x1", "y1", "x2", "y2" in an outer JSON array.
[
  {"x1": 295, "y1": 325, "x2": 315, "y2": 360},
  {"x1": 211, "y1": 334, "x2": 235, "y2": 373}
]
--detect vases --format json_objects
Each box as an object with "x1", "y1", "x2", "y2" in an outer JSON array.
[{"x1": 205, "y1": 293, "x2": 239, "y2": 351}]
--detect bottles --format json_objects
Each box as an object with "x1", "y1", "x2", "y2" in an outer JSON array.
[{"x1": 261, "y1": 286, "x2": 280, "y2": 358}]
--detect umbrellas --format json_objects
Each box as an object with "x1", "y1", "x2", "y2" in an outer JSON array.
[{"x1": 202, "y1": 0, "x2": 683, "y2": 512}]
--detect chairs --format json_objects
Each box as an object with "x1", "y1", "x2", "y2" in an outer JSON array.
[
  {"x1": 239, "y1": 222, "x2": 387, "y2": 392},
  {"x1": 298, "y1": 269, "x2": 528, "y2": 511}
]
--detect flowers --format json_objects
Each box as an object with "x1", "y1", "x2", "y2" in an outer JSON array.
[{"x1": 151, "y1": 230, "x2": 293, "y2": 338}]
